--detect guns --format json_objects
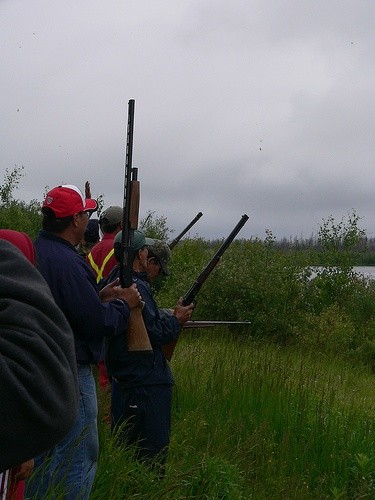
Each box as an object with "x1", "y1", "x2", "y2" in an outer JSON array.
[
  {"x1": 182, "y1": 320, "x2": 252, "y2": 328},
  {"x1": 160, "y1": 213, "x2": 250, "y2": 361},
  {"x1": 119, "y1": 99, "x2": 153, "y2": 352},
  {"x1": 170, "y1": 211, "x2": 203, "y2": 249}
]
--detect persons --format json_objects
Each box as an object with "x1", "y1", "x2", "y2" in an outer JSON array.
[
  {"x1": 85, "y1": 206, "x2": 123, "y2": 427},
  {"x1": 29, "y1": 186, "x2": 142, "y2": 500},
  {"x1": 0, "y1": 239, "x2": 78, "y2": 474},
  {"x1": 105, "y1": 229, "x2": 194, "y2": 479},
  {"x1": 75, "y1": 219, "x2": 104, "y2": 261},
  {"x1": 0, "y1": 229, "x2": 35, "y2": 500}
]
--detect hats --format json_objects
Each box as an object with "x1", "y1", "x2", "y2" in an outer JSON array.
[
  {"x1": 113, "y1": 230, "x2": 155, "y2": 249},
  {"x1": 99, "y1": 205, "x2": 123, "y2": 224},
  {"x1": 83, "y1": 219, "x2": 101, "y2": 242},
  {"x1": 41, "y1": 184, "x2": 98, "y2": 220},
  {"x1": 149, "y1": 238, "x2": 171, "y2": 276}
]
